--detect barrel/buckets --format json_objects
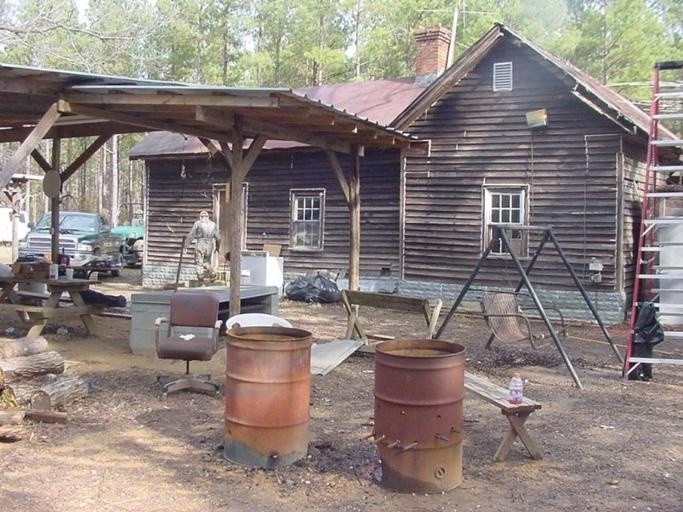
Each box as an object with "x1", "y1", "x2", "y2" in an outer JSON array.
[
  {"x1": 223, "y1": 326, "x2": 312, "y2": 471},
  {"x1": 373, "y1": 338, "x2": 463, "y2": 492}
]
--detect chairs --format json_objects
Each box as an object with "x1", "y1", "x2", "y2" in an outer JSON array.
[{"x1": 152, "y1": 293, "x2": 221, "y2": 393}]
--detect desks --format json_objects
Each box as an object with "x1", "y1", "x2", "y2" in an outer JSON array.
[{"x1": 0, "y1": 274, "x2": 101, "y2": 340}]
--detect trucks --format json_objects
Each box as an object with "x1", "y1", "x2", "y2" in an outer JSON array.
[
  {"x1": 0, "y1": 208, "x2": 34, "y2": 245},
  {"x1": 110, "y1": 201, "x2": 146, "y2": 266}
]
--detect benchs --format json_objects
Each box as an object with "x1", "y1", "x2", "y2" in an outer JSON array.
[{"x1": 460, "y1": 369, "x2": 543, "y2": 461}]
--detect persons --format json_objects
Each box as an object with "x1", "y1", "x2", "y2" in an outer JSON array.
[{"x1": 182, "y1": 211, "x2": 220, "y2": 288}]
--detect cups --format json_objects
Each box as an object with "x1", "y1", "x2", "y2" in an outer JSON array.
[
  {"x1": 66, "y1": 269, "x2": 73, "y2": 280},
  {"x1": 49, "y1": 264, "x2": 58, "y2": 279}
]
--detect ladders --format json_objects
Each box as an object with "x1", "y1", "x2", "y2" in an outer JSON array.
[{"x1": 621, "y1": 60, "x2": 683, "y2": 380}]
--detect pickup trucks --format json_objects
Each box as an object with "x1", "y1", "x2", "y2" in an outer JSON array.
[{"x1": 17, "y1": 209, "x2": 126, "y2": 281}]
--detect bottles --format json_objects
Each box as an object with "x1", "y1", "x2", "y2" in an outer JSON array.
[{"x1": 508, "y1": 371, "x2": 524, "y2": 404}]
